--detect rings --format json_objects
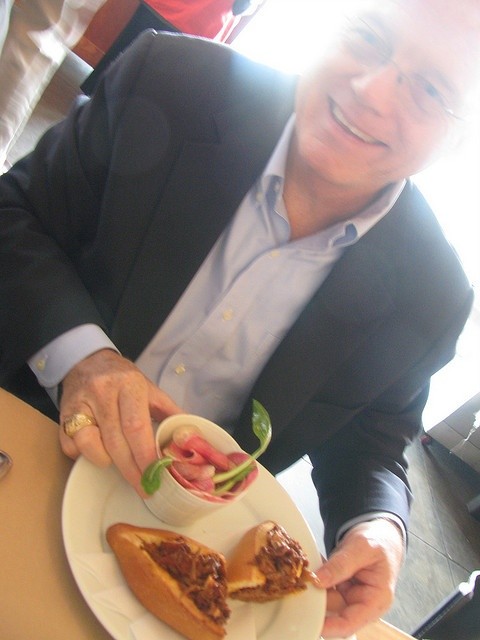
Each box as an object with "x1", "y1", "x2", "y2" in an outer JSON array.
[{"x1": 63, "y1": 412, "x2": 97, "y2": 438}]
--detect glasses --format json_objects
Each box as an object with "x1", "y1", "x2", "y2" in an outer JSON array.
[{"x1": 338, "y1": 13, "x2": 472, "y2": 129}]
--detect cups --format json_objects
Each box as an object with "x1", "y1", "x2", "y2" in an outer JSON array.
[{"x1": 140, "y1": 414, "x2": 249, "y2": 528}]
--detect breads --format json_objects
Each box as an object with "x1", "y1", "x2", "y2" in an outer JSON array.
[
  {"x1": 227, "y1": 521, "x2": 322, "y2": 603},
  {"x1": 107, "y1": 524, "x2": 227, "y2": 639}
]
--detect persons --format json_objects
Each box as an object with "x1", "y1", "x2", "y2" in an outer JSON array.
[
  {"x1": 0, "y1": 0, "x2": 109, "y2": 171},
  {"x1": 70, "y1": 0, "x2": 252, "y2": 114},
  {"x1": 0, "y1": 0, "x2": 479, "y2": 640}
]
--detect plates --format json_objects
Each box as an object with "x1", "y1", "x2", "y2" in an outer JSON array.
[{"x1": 61, "y1": 422, "x2": 327, "y2": 639}]
